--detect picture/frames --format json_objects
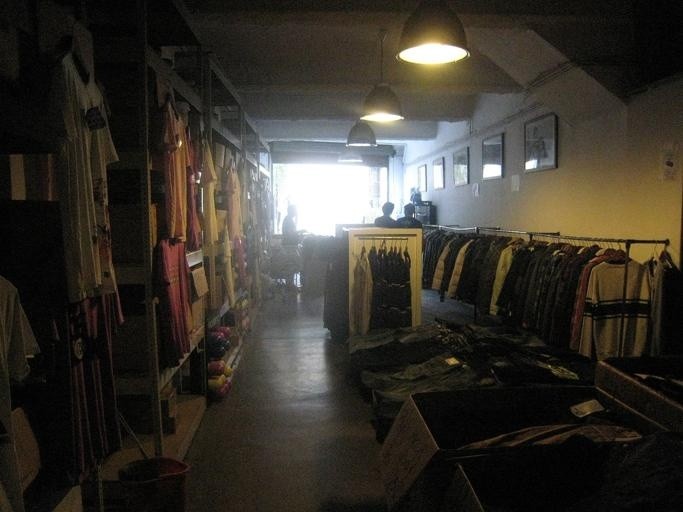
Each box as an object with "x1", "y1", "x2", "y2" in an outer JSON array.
[{"x1": 418, "y1": 112, "x2": 559, "y2": 192}]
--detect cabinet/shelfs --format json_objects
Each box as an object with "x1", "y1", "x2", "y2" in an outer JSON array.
[{"x1": 107, "y1": 0, "x2": 270, "y2": 478}]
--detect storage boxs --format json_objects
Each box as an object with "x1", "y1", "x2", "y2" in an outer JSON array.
[
  {"x1": 448, "y1": 440, "x2": 680, "y2": 512},
  {"x1": 595, "y1": 356, "x2": 681, "y2": 439},
  {"x1": 376, "y1": 385, "x2": 669, "y2": 510}
]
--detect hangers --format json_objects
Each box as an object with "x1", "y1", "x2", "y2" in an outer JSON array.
[
  {"x1": 533, "y1": 232, "x2": 672, "y2": 273},
  {"x1": 361, "y1": 238, "x2": 410, "y2": 258}
]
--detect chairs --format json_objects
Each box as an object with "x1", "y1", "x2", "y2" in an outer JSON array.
[{"x1": 77, "y1": 456, "x2": 193, "y2": 512}]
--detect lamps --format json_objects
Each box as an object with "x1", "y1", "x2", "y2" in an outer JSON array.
[{"x1": 346, "y1": 0, "x2": 472, "y2": 148}]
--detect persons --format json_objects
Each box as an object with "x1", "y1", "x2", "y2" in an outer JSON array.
[
  {"x1": 391, "y1": 204, "x2": 421, "y2": 227},
  {"x1": 279, "y1": 203, "x2": 307, "y2": 292},
  {"x1": 372, "y1": 201, "x2": 397, "y2": 227}
]
